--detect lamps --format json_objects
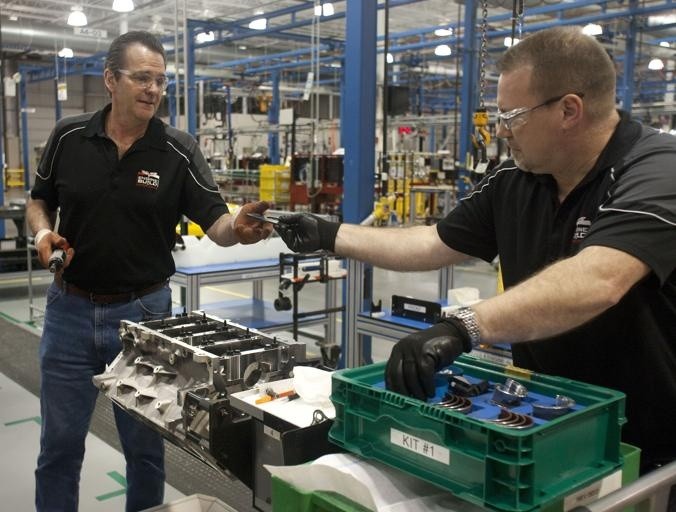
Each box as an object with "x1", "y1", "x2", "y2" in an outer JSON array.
[
  {"x1": 386, "y1": 22, "x2": 670, "y2": 71},
  {"x1": 57, "y1": 0, "x2": 335, "y2": 60}
]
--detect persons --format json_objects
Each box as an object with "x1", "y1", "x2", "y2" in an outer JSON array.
[
  {"x1": 271, "y1": 20, "x2": 676, "y2": 512},
  {"x1": 19, "y1": 31, "x2": 281, "y2": 511}
]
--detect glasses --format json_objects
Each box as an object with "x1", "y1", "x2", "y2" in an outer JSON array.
[
  {"x1": 497, "y1": 90, "x2": 582, "y2": 132},
  {"x1": 117, "y1": 69, "x2": 170, "y2": 91}
]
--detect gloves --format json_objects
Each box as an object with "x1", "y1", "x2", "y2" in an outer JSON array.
[
  {"x1": 230, "y1": 199, "x2": 273, "y2": 244},
  {"x1": 34, "y1": 227, "x2": 74, "y2": 272},
  {"x1": 273, "y1": 212, "x2": 341, "y2": 254},
  {"x1": 384, "y1": 316, "x2": 471, "y2": 401}
]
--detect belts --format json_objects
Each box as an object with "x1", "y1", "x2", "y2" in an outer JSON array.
[{"x1": 50, "y1": 273, "x2": 167, "y2": 308}]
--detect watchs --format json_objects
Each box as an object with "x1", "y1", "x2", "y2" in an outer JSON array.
[{"x1": 448, "y1": 306, "x2": 480, "y2": 349}]
